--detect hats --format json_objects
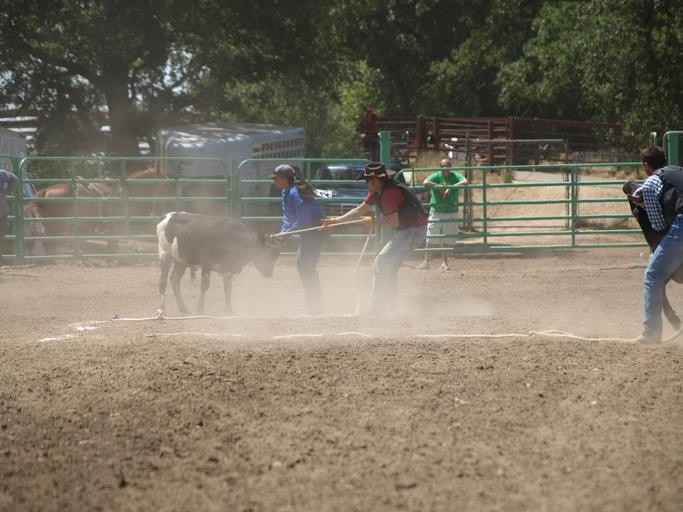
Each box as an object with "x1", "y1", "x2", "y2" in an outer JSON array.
[
  {"x1": 268, "y1": 164, "x2": 296, "y2": 180},
  {"x1": 357, "y1": 163, "x2": 398, "y2": 181}
]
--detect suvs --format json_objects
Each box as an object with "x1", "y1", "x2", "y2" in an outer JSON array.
[{"x1": 302, "y1": 161, "x2": 404, "y2": 249}]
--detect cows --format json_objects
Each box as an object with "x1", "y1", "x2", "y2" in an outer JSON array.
[{"x1": 156, "y1": 212, "x2": 288, "y2": 317}]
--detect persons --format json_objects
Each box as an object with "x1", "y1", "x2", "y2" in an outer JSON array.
[
  {"x1": 267, "y1": 161, "x2": 328, "y2": 309},
  {"x1": 321, "y1": 162, "x2": 428, "y2": 319},
  {"x1": 418, "y1": 159, "x2": 468, "y2": 271},
  {"x1": 630, "y1": 146, "x2": 683, "y2": 343},
  {"x1": 0, "y1": 166, "x2": 20, "y2": 239},
  {"x1": 362, "y1": 105, "x2": 378, "y2": 157}
]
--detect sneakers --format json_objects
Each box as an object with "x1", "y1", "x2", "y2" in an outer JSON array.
[
  {"x1": 633, "y1": 335, "x2": 662, "y2": 344},
  {"x1": 420, "y1": 261, "x2": 432, "y2": 270},
  {"x1": 441, "y1": 262, "x2": 451, "y2": 271}
]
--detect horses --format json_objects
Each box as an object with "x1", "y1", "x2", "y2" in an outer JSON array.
[{"x1": 22, "y1": 161, "x2": 176, "y2": 266}]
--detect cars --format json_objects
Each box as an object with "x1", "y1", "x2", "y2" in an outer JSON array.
[{"x1": 1, "y1": 170, "x2": 36, "y2": 261}]
